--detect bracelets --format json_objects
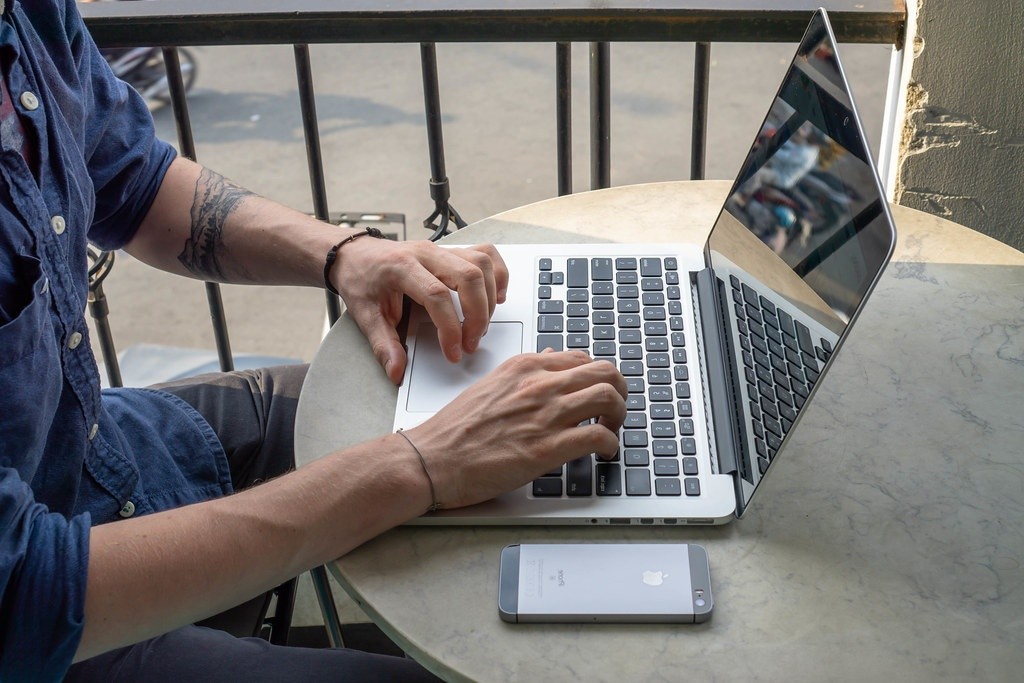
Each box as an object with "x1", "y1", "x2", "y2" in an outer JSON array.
[
  {"x1": 323, "y1": 226, "x2": 389, "y2": 296},
  {"x1": 396, "y1": 428, "x2": 443, "y2": 512}
]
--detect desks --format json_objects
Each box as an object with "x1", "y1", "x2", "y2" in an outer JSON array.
[{"x1": 293, "y1": 179, "x2": 1024, "y2": 683}]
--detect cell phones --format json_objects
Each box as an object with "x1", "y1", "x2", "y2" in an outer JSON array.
[{"x1": 499, "y1": 543, "x2": 715, "y2": 624}]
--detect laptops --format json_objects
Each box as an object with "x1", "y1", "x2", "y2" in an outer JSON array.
[{"x1": 391, "y1": 6, "x2": 897, "y2": 524}]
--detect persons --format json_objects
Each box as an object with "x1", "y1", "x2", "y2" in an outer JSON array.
[
  {"x1": 731, "y1": 120, "x2": 830, "y2": 254},
  {"x1": 0, "y1": 1, "x2": 629, "y2": 682}
]
